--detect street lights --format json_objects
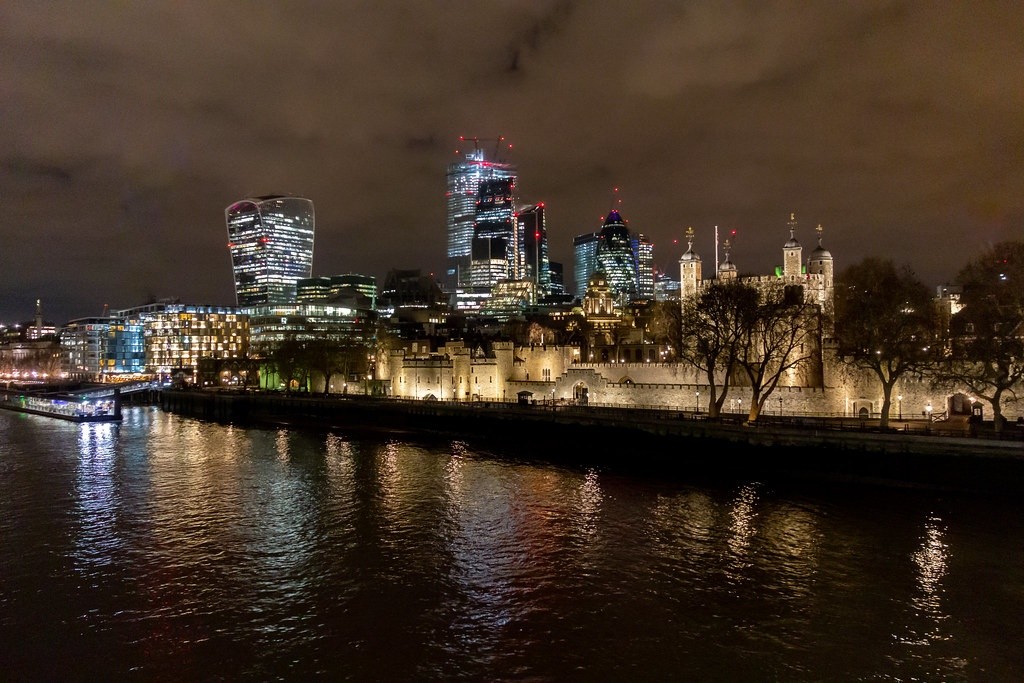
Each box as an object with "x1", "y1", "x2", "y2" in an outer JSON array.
[
  {"x1": 695, "y1": 390, "x2": 700, "y2": 412},
  {"x1": 737, "y1": 398, "x2": 742, "y2": 414},
  {"x1": 552, "y1": 388, "x2": 555, "y2": 400},
  {"x1": 897, "y1": 395, "x2": 903, "y2": 419}
]
